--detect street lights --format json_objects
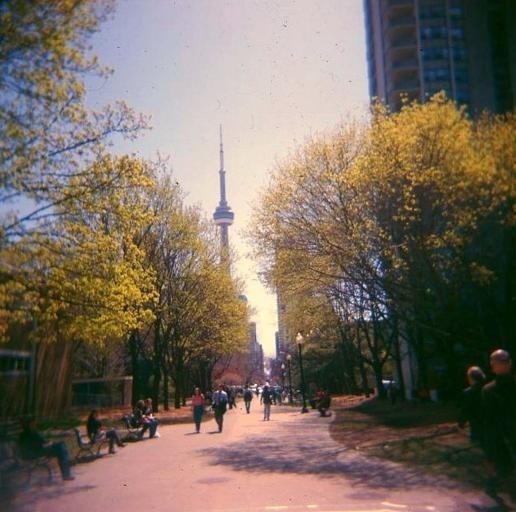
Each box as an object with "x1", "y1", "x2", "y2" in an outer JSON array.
[
  {"x1": 296, "y1": 332, "x2": 309, "y2": 413},
  {"x1": 281, "y1": 354, "x2": 292, "y2": 403}
]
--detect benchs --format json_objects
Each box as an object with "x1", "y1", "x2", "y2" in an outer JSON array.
[{"x1": 5, "y1": 412, "x2": 134, "y2": 491}]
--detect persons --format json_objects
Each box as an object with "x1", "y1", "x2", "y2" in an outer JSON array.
[
  {"x1": 457, "y1": 349, "x2": 516, "y2": 512},
  {"x1": 192, "y1": 383, "x2": 228, "y2": 433},
  {"x1": 228, "y1": 385, "x2": 283, "y2": 422},
  {"x1": 130, "y1": 397, "x2": 161, "y2": 439},
  {"x1": 86, "y1": 408, "x2": 127, "y2": 454},
  {"x1": 309, "y1": 386, "x2": 331, "y2": 416},
  {"x1": 18, "y1": 416, "x2": 76, "y2": 481}
]
